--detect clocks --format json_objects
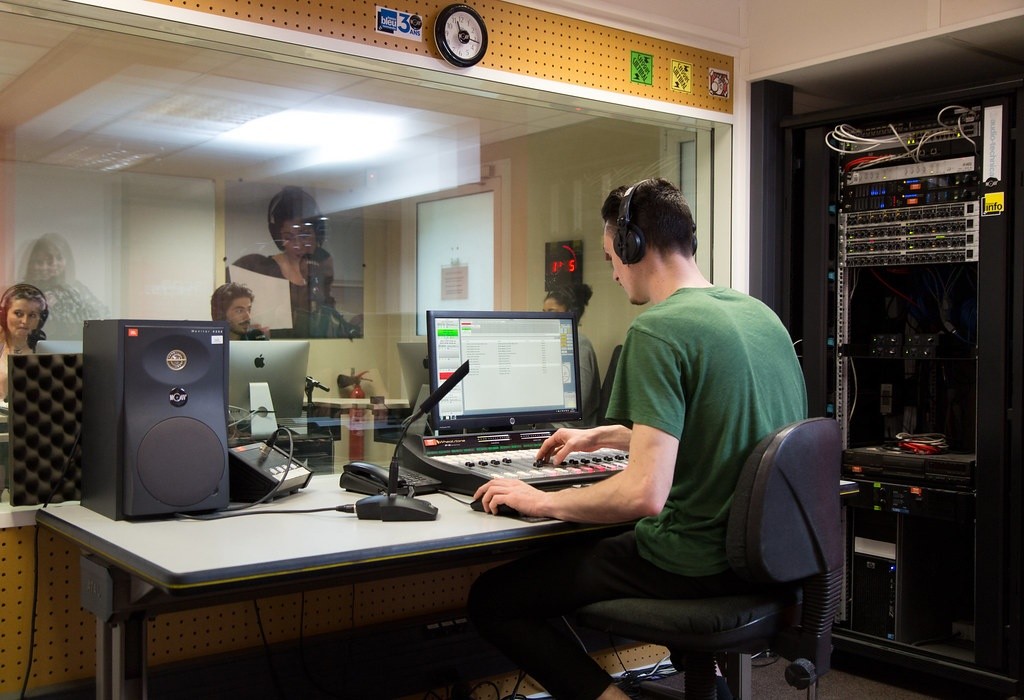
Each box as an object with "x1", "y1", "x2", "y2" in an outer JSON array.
[{"x1": 434, "y1": 4, "x2": 488, "y2": 68}]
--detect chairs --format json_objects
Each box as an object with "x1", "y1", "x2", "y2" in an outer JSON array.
[{"x1": 572, "y1": 417, "x2": 844, "y2": 700}]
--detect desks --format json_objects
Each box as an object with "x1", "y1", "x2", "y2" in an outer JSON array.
[{"x1": 35, "y1": 474, "x2": 646, "y2": 700}]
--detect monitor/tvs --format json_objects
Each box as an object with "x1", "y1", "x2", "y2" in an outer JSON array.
[
  {"x1": 426, "y1": 308, "x2": 585, "y2": 430},
  {"x1": 229, "y1": 339, "x2": 309, "y2": 440}
]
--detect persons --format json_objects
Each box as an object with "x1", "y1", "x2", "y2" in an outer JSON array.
[
  {"x1": 491, "y1": 280, "x2": 601, "y2": 431},
  {"x1": 0, "y1": 286, "x2": 50, "y2": 405},
  {"x1": 210, "y1": 282, "x2": 255, "y2": 342},
  {"x1": 225, "y1": 186, "x2": 338, "y2": 338},
  {"x1": 465, "y1": 180, "x2": 837, "y2": 699},
  {"x1": 18, "y1": 231, "x2": 111, "y2": 341}
]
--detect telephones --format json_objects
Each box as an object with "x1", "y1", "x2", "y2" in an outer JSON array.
[{"x1": 340, "y1": 461, "x2": 442, "y2": 497}]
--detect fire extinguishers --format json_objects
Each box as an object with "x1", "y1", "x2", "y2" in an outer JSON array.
[{"x1": 337, "y1": 370, "x2": 375, "y2": 461}]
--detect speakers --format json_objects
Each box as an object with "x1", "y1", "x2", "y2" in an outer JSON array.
[{"x1": 82, "y1": 318, "x2": 230, "y2": 522}]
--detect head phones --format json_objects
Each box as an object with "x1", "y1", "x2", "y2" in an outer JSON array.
[
  {"x1": 548, "y1": 284, "x2": 583, "y2": 322},
  {"x1": 213, "y1": 283, "x2": 237, "y2": 324},
  {"x1": 613, "y1": 178, "x2": 698, "y2": 264},
  {"x1": 267, "y1": 188, "x2": 326, "y2": 250},
  {"x1": 0, "y1": 283, "x2": 49, "y2": 331}
]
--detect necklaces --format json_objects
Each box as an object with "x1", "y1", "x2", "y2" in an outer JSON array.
[{"x1": 10, "y1": 340, "x2": 28, "y2": 354}]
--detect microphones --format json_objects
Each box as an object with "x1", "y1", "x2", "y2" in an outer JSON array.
[
  {"x1": 355, "y1": 360, "x2": 471, "y2": 522},
  {"x1": 305, "y1": 376, "x2": 330, "y2": 393}
]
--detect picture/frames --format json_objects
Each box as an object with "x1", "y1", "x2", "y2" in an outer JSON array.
[{"x1": 214, "y1": 178, "x2": 364, "y2": 339}]
